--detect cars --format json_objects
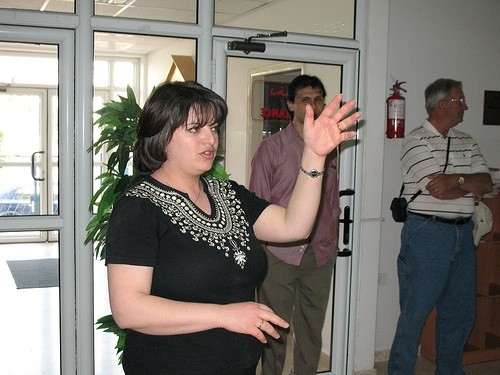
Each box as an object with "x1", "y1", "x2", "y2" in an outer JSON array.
[{"x1": 0, "y1": 187, "x2": 61, "y2": 215}]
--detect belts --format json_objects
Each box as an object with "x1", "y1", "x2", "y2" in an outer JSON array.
[{"x1": 408, "y1": 212, "x2": 473, "y2": 225}]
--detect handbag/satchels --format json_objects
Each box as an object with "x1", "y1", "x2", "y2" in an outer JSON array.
[{"x1": 390, "y1": 197, "x2": 408, "y2": 222}]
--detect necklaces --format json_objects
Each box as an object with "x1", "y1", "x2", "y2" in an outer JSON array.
[{"x1": 194, "y1": 192, "x2": 201, "y2": 203}]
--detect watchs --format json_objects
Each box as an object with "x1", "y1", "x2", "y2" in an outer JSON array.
[
  {"x1": 457, "y1": 175, "x2": 465, "y2": 189},
  {"x1": 300, "y1": 166, "x2": 324, "y2": 179}
]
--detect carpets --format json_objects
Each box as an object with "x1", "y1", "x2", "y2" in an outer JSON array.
[{"x1": 6, "y1": 258, "x2": 59, "y2": 289}]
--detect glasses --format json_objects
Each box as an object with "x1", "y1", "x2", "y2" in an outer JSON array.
[{"x1": 448, "y1": 95, "x2": 466, "y2": 105}]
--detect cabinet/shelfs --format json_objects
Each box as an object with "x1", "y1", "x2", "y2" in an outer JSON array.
[{"x1": 421, "y1": 192, "x2": 500, "y2": 365}]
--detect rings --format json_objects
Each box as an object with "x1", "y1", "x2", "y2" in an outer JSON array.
[
  {"x1": 258, "y1": 321, "x2": 264, "y2": 329},
  {"x1": 337, "y1": 124, "x2": 342, "y2": 131},
  {"x1": 434, "y1": 188, "x2": 438, "y2": 192}
]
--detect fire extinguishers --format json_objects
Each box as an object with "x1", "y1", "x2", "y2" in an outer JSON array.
[{"x1": 387, "y1": 80, "x2": 407, "y2": 139}]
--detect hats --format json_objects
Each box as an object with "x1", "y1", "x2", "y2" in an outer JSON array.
[{"x1": 473, "y1": 200, "x2": 493, "y2": 246}]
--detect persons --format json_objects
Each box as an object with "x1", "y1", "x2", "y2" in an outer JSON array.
[
  {"x1": 387, "y1": 77, "x2": 493, "y2": 375},
  {"x1": 248, "y1": 74, "x2": 344, "y2": 375},
  {"x1": 104, "y1": 81, "x2": 362, "y2": 375}
]
